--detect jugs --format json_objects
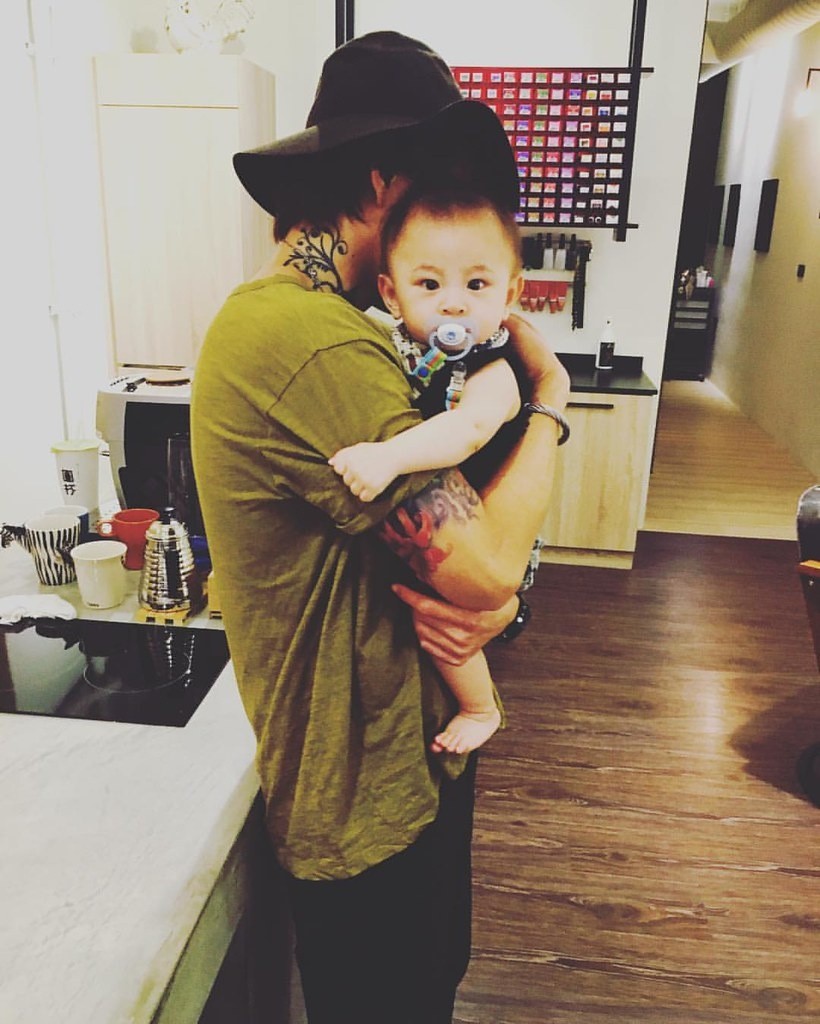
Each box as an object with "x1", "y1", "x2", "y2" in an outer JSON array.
[{"x1": 139, "y1": 507, "x2": 207, "y2": 612}]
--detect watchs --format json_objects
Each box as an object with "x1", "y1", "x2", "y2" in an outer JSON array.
[{"x1": 496, "y1": 595, "x2": 531, "y2": 642}]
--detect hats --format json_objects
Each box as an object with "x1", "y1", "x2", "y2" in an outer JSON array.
[{"x1": 232, "y1": 30, "x2": 520, "y2": 216}]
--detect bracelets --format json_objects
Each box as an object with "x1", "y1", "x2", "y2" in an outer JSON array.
[{"x1": 522, "y1": 403, "x2": 570, "y2": 446}]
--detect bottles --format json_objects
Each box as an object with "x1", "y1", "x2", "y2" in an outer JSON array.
[
  {"x1": 596, "y1": 319, "x2": 615, "y2": 370},
  {"x1": 537, "y1": 233, "x2": 575, "y2": 271}
]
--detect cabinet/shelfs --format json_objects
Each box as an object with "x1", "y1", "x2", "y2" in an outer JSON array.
[
  {"x1": 665, "y1": 284, "x2": 715, "y2": 382},
  {"x1": 539, "y1": 396, "x2": 663, "y2": 571},
  {"x1": 151, "y1": 781, "x2": 291, "y2": 1024},
  {"x1": 91, "y1": 52, "x2": 277, "y2": 383}
]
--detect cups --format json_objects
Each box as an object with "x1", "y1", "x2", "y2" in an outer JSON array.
[
  {"x1": 70, "y1": 540, "x2": 127, "y2": 609},
  {"x1": 97, "y1": 509, "x2": 159, "y2": 570},
  {"x1": 24, "y1": 506, "x2": 89, "y2": 585},
  {"x1": 50, "y1": 439, "x2": 99, "y2": 513}
]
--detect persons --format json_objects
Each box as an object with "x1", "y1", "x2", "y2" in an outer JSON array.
[
  {"x1": 190, "y1": 30, "x2": 570, "y2": 1024},
  {"x1": 329, "y1": 184, "x2": 543, "y2": 756}
]
